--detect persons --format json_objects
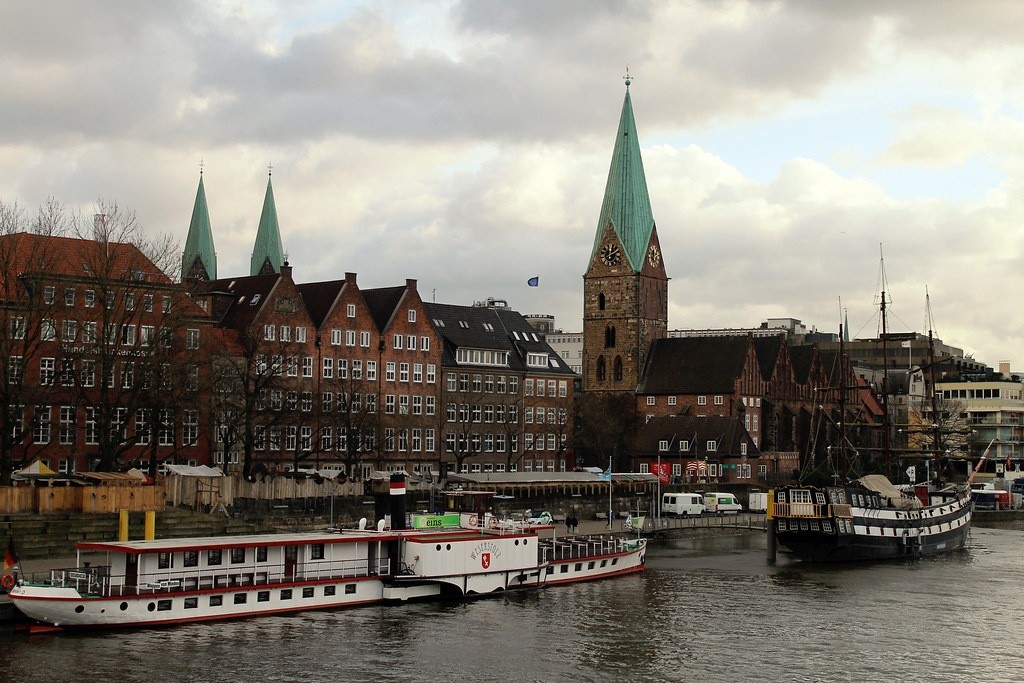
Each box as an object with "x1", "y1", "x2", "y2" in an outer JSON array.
[
  {"x1": 605, "y1": 509, "x2": 613, "y2": 529},
  {"x1": 565, "y1": 515, "x2": 579, "y2": 533}
]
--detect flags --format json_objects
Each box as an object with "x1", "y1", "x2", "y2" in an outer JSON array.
[
  {"x1": 597, "y1": 467, "x2": 610, "y2": 481},
  {"x1": 3, "y1": 534, "x2": 18, "y2": 572},
  {"x1": 527, "y1": 276, "x2": 538, "y2": 287},
  {"x1": 660, "y1": 466, "x2": 669, "y2": 484}
]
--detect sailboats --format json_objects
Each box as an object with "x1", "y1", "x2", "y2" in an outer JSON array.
[{"x1": 771, "y1": 242, "x2": 972, "y2": 562}]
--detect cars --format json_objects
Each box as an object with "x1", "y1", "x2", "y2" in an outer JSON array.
[{"x1": 522, "y1": 510, "x2": 554, "y2": 525}]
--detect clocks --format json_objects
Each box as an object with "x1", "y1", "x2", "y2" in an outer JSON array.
[
  {"x1": 600, "y1": 244, "x2": 621, "y2": 265},
  {"x1": 649, "y1": 246, "x2": 660, "y2": 268}
]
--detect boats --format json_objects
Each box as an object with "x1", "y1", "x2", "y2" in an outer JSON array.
[
  {"x1": 0, "y1": 574, "x2": 28, "y2": 603},
  {"x1": 9, "y1": 474, "x2": 647, "y2": 626}
]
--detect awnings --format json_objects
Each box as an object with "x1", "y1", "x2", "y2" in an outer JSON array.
[{"x1": 686, "y1": 458, "x2": 707, "y2": 470}]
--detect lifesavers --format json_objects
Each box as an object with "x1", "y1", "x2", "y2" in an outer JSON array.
[
  {"x1": 1, "y1": 573, "x2": 14, "y2": 588},
  {"x1": 641, "y1": 554, "x2": 645, "y2": 564},
  {"x1": 469, "y1": 515, "x2": 478, "y2": 526},
  {"x1": 488, "y1": 517, "x2": 497, "y2": 529}
]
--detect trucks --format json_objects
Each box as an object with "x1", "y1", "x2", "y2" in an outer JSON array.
[
  {"x1": 749, "y1": 493, "x2": 768, "y2": 514},
  {"x1": 661, "y1": 493, "x2": 706, "y2": 519},
  {"x1": 704, "y1": 493, "x2": 742, "y2": 515}
]
